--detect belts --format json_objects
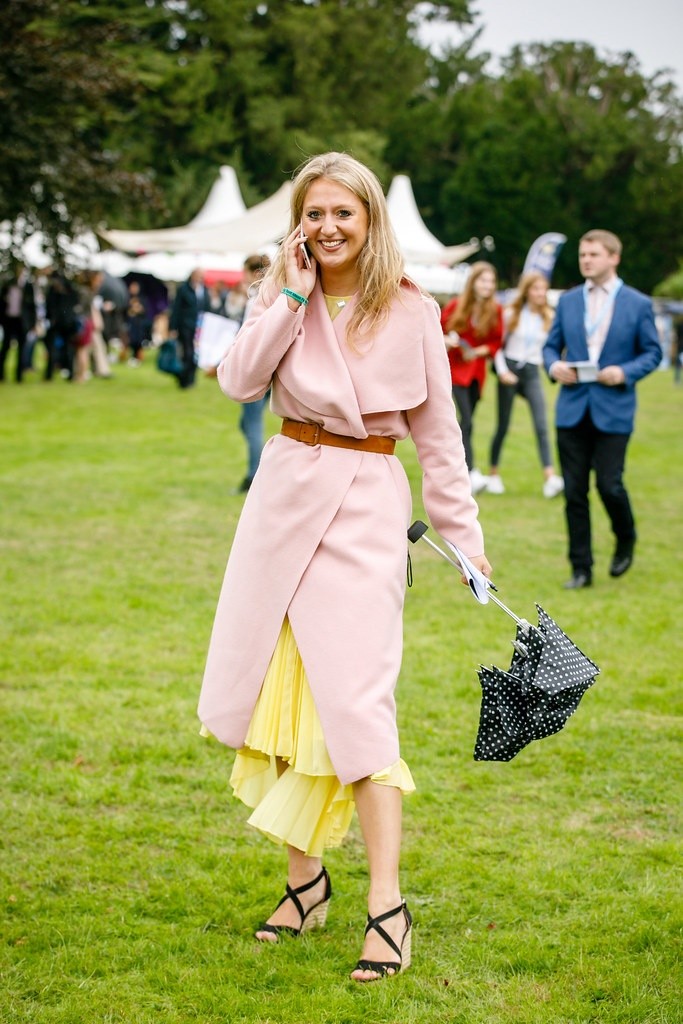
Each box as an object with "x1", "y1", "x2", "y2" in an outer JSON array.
[{"x1": 280, "y1": 417, "x2": 396, "y2": 455}]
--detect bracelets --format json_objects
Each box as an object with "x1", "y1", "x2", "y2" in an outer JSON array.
[{"x1": 280, "y1": 288, "x2": 309, "y2": 306}]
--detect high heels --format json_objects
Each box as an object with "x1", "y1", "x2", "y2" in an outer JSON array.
[
  {"x1": 253, "y1": 866, "x2": 332, "y2": 943},
  {"x1": 349, "y1": 897, "x2": 413, "y2": 983}
]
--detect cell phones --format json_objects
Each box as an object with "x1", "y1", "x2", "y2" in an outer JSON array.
[{"x1": 298, "y1": 217, "x2": 312, "y2": 270}]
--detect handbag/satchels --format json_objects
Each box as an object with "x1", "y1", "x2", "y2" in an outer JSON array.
[
  {"x1": 490, "y1": 305, "x2": 520, "y2": 374},
  {"x1": 155, "y1": 340, "x2": 184, "y2": 375}
]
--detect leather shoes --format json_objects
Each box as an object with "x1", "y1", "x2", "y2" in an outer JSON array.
[
  {"x1": 564, "y1": 572, "x2": 593, "y2": 589},
  {"x1": 608, "y1": 534, "x2": 637, "y2": 577}
]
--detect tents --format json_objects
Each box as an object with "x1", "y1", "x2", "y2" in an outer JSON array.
[{"x1": 97, "y1": 165, "x2": 472, "y2": 295}]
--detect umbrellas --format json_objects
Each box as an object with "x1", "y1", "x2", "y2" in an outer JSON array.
[{"x1": 407, "y1": 520, "x2": 601, "y2": 764}]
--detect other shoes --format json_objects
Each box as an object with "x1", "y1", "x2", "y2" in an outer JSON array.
[
  {"x1": 487, "y1": 476, "x2": 505, "y2": 494},
  {"x1": 543, "y1": 475, "x2": 564, "y2": 499},
  {"x1": 469, "y1": 469, "x2": 487, "y2": 494}
]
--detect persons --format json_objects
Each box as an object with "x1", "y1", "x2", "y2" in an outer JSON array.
[
  {"x1": 197, "y1": 151, "x2": 492, "y2": 982},
  {"x1": 542, "y1": 229, "x2": 664, "y2": 589},
  {"x1": 0, "y1": 253, "x2": 565, "y2": 499}
]
